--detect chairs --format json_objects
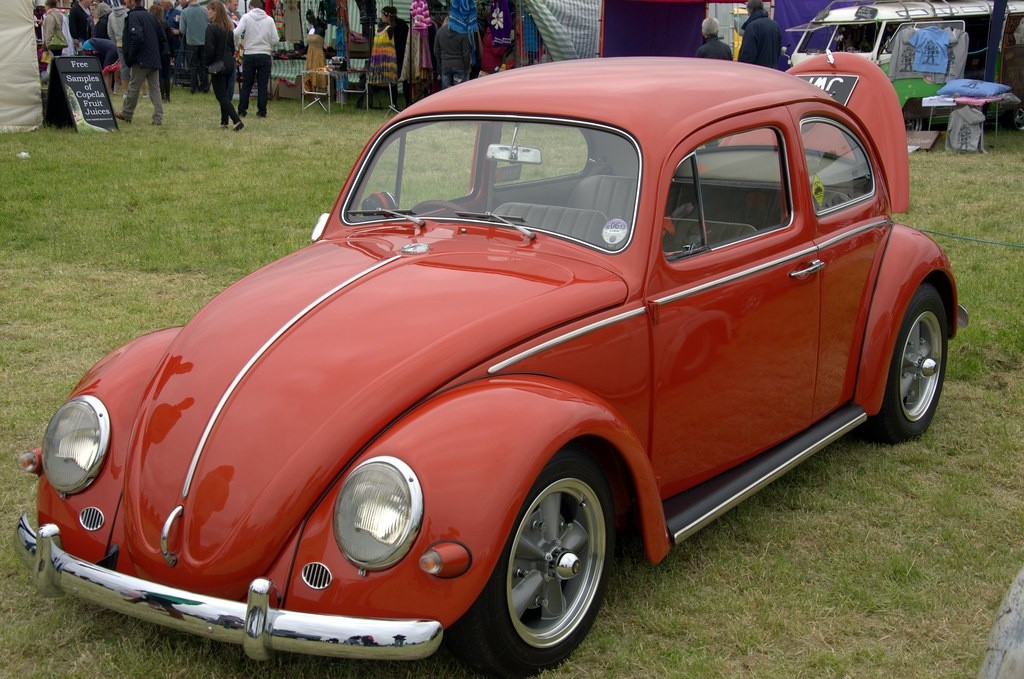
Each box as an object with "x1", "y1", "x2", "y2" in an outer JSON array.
[
  {"x1": 662, "y1": 217, "x2": 756, "y2": 253},
  {"x1": 488, "y1": 202, "x2": 607, "y2": 250}
]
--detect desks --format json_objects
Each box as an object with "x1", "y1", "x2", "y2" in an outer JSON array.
[
  {"x1": 301, "y1": 69, "x2": 369, "y2": 115},
  {"x1": 922, "y1": 95, "x2": 1001, "y2": 154}
]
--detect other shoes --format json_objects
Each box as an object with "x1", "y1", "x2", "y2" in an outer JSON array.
[
  {"x1": 151, "y1": 121, "x2": 161, "y2": 125},
  {"x1": 142, "y1": 93, "x2": 149, "y2": 98},
  {"x1": 256, "y1": 111, "x2": 266, "y2": 117},
  {"x1": 123, "y1": 93, "x2": 127, "y2": 98},
  {"x1": 115, "y1": 111, "x2": 132, "y2": 122},
  {"x1": 221, "y1": 126, "x2": 228, "y2": 129},
  {"x1": 233, "y1": 122, "x2": 244, "y2": 131},
  {"x1": 238, "y1": 111, "x2": 247, "y2": 117}
]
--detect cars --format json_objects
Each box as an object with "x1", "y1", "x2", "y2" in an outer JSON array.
[{"x1": 15, "y1": 51, "x2": 970, "y2": 679}]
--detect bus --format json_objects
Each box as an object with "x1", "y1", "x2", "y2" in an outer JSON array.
[{"x1": 780, "y1": 0, "x2": 1024, "y2": 133}]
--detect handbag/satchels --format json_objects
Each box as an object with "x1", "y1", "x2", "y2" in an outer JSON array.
[
  {"x1": 47, "y1": 14, "x2": 68, "y2": 49},
  {"x1": 208, "y1": 61, "x2": 226, "y2": 74},
  {"x1": 40, "y1": 51, "x2": 50, "y2": 63}
]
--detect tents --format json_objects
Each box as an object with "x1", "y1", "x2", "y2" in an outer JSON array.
[{"x1": 0, "y1": 0, "x2": 43, "y2": 134}]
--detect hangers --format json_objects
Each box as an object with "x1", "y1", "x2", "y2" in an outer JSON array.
[{"x1": 949, "y1": 21, "x2": 954, "y2": 30}]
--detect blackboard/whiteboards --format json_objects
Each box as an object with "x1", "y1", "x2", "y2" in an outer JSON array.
[{"x1": 42, "y1": 56, "x2": 120, "y2": 133}]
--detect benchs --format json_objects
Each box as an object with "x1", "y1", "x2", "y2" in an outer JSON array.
[{"x1": 567, "y1": 175, "x2": 850, "y2": 235}]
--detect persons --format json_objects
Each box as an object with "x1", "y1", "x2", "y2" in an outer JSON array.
[
  {"x1": 738, "y1": 0, "x2": 781, "y2": 67},
  {"x1": 203, "y1": 0, "x2": 244, "y2": 132},
  {"x1": 33, "y1": 0, "x2": 121, "y2": 96},
  {"x1": 381, "y1": 6, "x2": 412, "y2": 111},
  {"x1": 115, "y1": 0, "x2": 170, "y2": 125},
  {"x1": 367, "y1": 22, "x2": 398, "y2": 86},
  {"x1": 433, "y1": 13, "x2": 470, "y2": 90},
  {"x1": 107, "y1": 0, "x2": 150, "y2": 99},
  {"x1": 147, "y1": 0, "x2": 188, "y2": 102},
  {"x1": 694, "y1": 16, "x2": 733, "y2": 60},
  {"x1": 304, "y1": 24, "x2": 327, "y2": 92},
  {"x1": 223, "y1": 0, "x2": 280, "y2": 118},
  {"x1": 481, "y1": 25, "x2": 510, "y2": 74},
  {"x1": 179, "y1": 0, "x2": 210, "y2": 95}
]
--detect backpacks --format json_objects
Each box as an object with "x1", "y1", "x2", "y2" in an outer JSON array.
[{"x1": 945, "y1": 106, "x2": 985, "y2": 153}]
